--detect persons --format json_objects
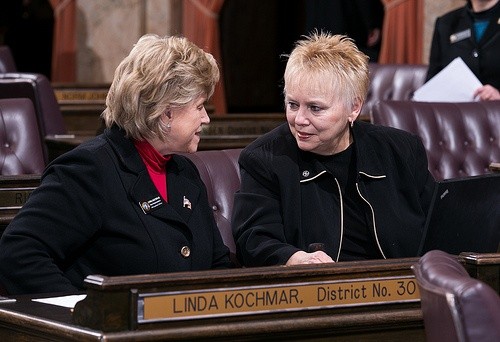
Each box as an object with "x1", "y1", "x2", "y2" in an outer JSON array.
[
  {"x1": 423, "y1": 0, "x2": 500, "y2": 101},
  {"x1": 1, "y1": 33, "x2": 240, "y2": 298},
  {"x1": 228, "y1": 31, "x2": 441, "y2": 267}
]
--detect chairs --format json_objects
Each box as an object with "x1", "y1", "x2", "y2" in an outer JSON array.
[
  {"x1": 1, "y1": 72, "x2": 59, "y2": 177},
  {"x1": 357, "y1": 62, "x2": 431, "y2": 116},
  {"x1": 179, "y1": 148, "x2": 245, "y2": 253},
  {"x1": 411, "y1": 251, "x2": 500, "y2": 342},
  {"x1": 369, "y1": 97, "x2": 500, "y2": 178}
]
